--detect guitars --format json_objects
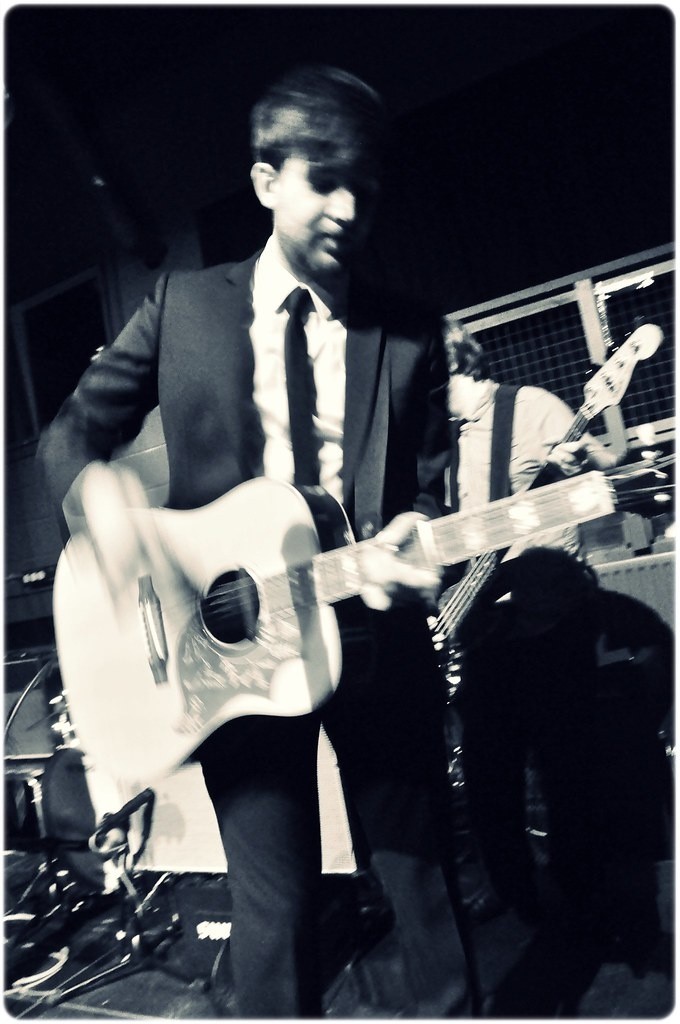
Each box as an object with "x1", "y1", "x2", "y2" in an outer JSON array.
[
  {"x1": 424, "y1": 314, "x2": 662, "y2": 707},
  {"x1": 48, "y1": 472, "x2": 616, "y2": 791}
]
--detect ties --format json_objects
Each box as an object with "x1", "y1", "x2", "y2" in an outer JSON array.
[
  {"x1": 448, "y1": 416, "x2": 468, "y2": 512},
  {"x1": 283, "y1": 288, "x2": 322, "y2": 488}
]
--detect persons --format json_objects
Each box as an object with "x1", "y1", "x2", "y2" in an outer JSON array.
[
  {"x1": 37, "y1": 66, "x2": 470, "y2": 1018},
  {"x1": 422, "y1": 318, "x2": 587, "y2": 927}
]
